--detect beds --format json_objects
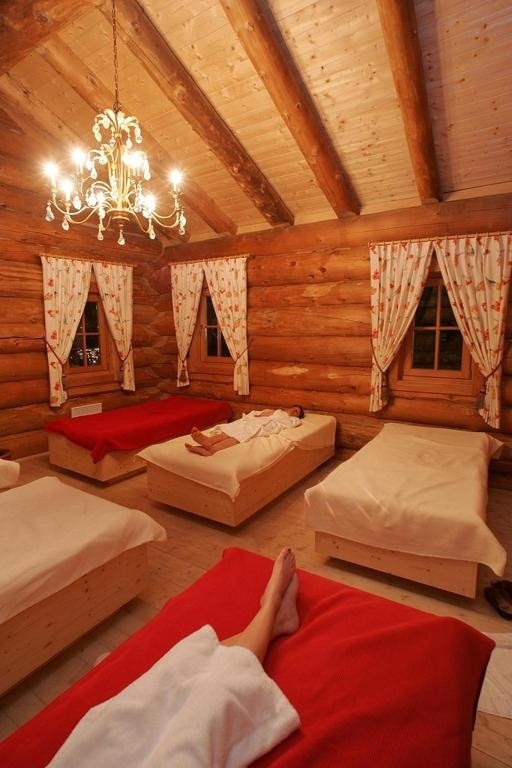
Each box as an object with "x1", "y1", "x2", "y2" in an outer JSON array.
[
  {"x1": 139, "y1": 408, "x2": 339, "y2": 529},
  {"x1": 0, "y1": 475, "x2": 169, "y2": 699},
  {"x1": 43, "y1": 393, "x2": 233, "y2": 485},
  {"x1": 302, "y1": 421, "x2": 511, "y2": 606},
  {"x1": 0, "y1": 546, "x2": 497, "y2": 765}
]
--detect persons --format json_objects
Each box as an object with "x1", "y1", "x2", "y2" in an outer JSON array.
[
  {"x1": 41, "y1": 544, "x2": 302, "y2": 767},
  {"x1": 184, "y1": 402, "x2": 305, "y2": 456}
]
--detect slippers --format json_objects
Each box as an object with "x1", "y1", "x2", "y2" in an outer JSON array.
[{"x1": 483, "y1": 578, "x2": 511, "y2": 620}]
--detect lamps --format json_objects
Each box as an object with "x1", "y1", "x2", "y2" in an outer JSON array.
[{"x1": 41, "y1": 0, "x2": 188, "y2": 246}]
